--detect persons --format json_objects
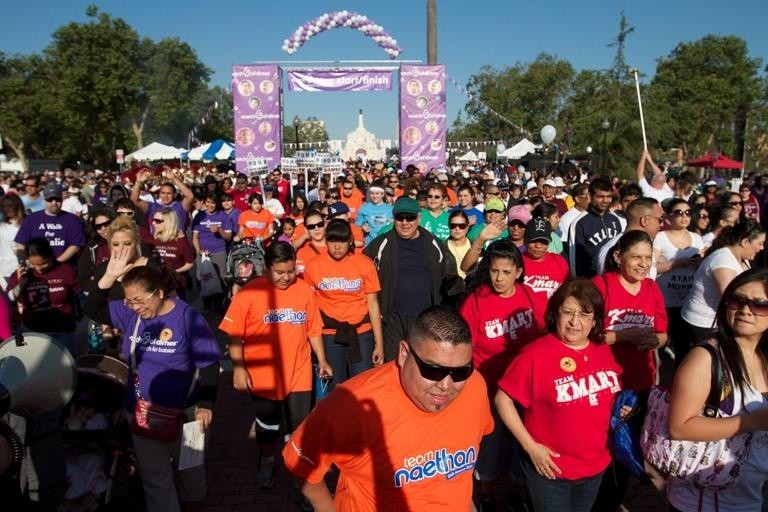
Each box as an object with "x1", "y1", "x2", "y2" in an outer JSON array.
[{"x1": 0, "y1": 152, "x2": 768, "y2": 512}]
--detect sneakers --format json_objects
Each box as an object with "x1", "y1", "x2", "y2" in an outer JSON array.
[
  {"x1": 291, "y1": 487, "x2": 312, "y2": 510},
  {"x1": 256, "y1": 456, "x2": 276, "y2": 489}
]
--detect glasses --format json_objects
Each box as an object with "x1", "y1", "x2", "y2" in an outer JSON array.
[
  {"x1": 47, "y1": 198, "x2": 61, "y2": 202},
  {"x1": 325, "y1": 194, "x2": 339, "y2": 200},
  {"x1": 344, "y1": 187, "x2": 352, "y2": 190},
  {"x1": 450, "y1": 223, "x2": 468, "y2": 229},
  {"x1": 488, "y1": 192, "x2": 500, "y2": 196},
  {"x1": 394, "y1": 214, "x2": 416, "y2": 221},
  {"x1": 648, "y1": 214, "x2": 663, "y2": 224},
  {"x1": 671, "y1": 209, "x2": 692, "y2": 216},
  {"x1": 124, "y1": 289, "x2": 157, "y2": 306},
  {"x1": 729, "y1": 201, "x2": 744, "y2": 206},
  {"x1": 306, "y1": 220, "x2": 324, "y2": 230},
  {"x1": 119, "y1": 212, "x2": 134, "y2": 217},
  {"x1": 96, "y1": 220, "x2": 112, "y2": 230},
  {"x1": 727, "y1": 293, "x2": 768, "y2": 316},
  {"x1": 410, "y1": 192, "x2": 417, "y2": 197},
  {"x1": 697, "y1": 215, "x2": 707, "y2": 221},
  {"x1": 152, "y1": 218, "x2": 164, "y2": 223},
  {"x1": 427, "y1": 195, "x2": 440, "y2": 199},
  {"x1": 408, "y1": 343, "x2": 474, "y2": 382}
]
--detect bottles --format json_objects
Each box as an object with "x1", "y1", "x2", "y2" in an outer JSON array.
[
  {"x1": 255, "y1": 234, "x2": 261, "y2": 244},
  {"x1": 86, "y1": 321, "x2": 105, "y2": 352}
]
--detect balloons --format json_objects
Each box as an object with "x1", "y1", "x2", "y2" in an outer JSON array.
[
  {"x1": 540, "y1": 125, "x2": 556, "y2": 144},
  {"x1": 281, "y1": 10, "x2": 403, "y2": 60}
]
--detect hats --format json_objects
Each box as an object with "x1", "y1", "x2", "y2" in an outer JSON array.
[
  {"x1": 327, "y1": 201, "x2": 349, "y2": 220},
  {"x1": 44, "y1": 183, "x2": 61, "y2": 199},
  {"x1": 526, "y1": 176, "x2": 566, "y2": 191},
  {"x1": 484, "y1": 199, "x2": 552, "y2": 243},
  {"x1": 394, "y1": 198, "x2": 419, "y2": 213},
  {"x1": 438, "y1": 174, "x2": 448, "y2": 182}
]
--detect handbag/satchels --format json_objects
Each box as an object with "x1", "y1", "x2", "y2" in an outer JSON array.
[
  {"x1": 130, "y1": 399, "x2": 182, "y2": 441},
  {"x1": 640, "y1": 384, "x2": 754, "y2": 491}
]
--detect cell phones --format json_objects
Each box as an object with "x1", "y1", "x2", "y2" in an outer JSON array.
[
  {"x1": 691, "y1": 254, "x2": 700, "y2": 258},
  {"x1": 317, "y1": 368, "x2": 333, "y2": 380}
]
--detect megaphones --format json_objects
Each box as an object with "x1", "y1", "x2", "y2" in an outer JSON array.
[{"x1": 0, "y1": 331, "x2": 79, "y2": 419}]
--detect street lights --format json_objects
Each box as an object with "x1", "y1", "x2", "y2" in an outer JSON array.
[
  {"x1": 601, "y1": 118, "x2": 611, "y2": 175},
  {"x1": 292, "y1": 114, "x2": 301, "y2": 150}
]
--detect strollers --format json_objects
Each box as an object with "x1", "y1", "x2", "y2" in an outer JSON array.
[
  {"x1": 43, "y1": 347, "x2": 133, "y2": 510},
  {"x1": 224, "y1": 235, "x2": 266, "y2": 309}
]
